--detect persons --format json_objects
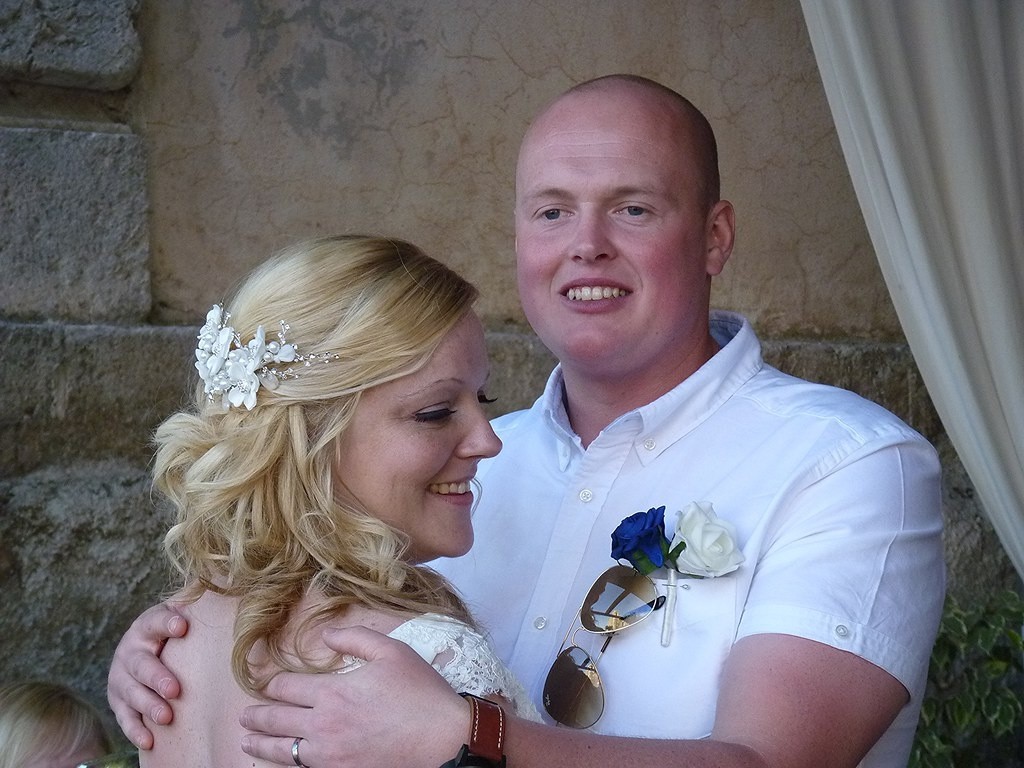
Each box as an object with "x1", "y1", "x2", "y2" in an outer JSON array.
[
  {"x1": 107, "y1": 74, "x2": 946, "y2": 768},
  {"x1": 0, "y1": 679, "x2": 125, "y2": 768},
  {"x1": 138, "y1": 234, "x2": 545, "y2": 768}
]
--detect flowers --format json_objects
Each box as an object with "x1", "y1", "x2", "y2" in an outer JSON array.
[{"x1": 600, "y1": 500, "x2": 747, "y2": 615}]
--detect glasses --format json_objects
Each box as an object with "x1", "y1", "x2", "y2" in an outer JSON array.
[{"x1": 542, "y1": 565, "x2": 658, "y2": 732}]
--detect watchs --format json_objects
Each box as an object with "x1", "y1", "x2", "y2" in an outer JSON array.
[{"x1": 440, "y1": 691, "x2": 507, "y2": 768}]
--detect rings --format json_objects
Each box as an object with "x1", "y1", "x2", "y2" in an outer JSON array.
[{"x1": 292, "y1": 737, "x2": 309, "y2": 768}]
